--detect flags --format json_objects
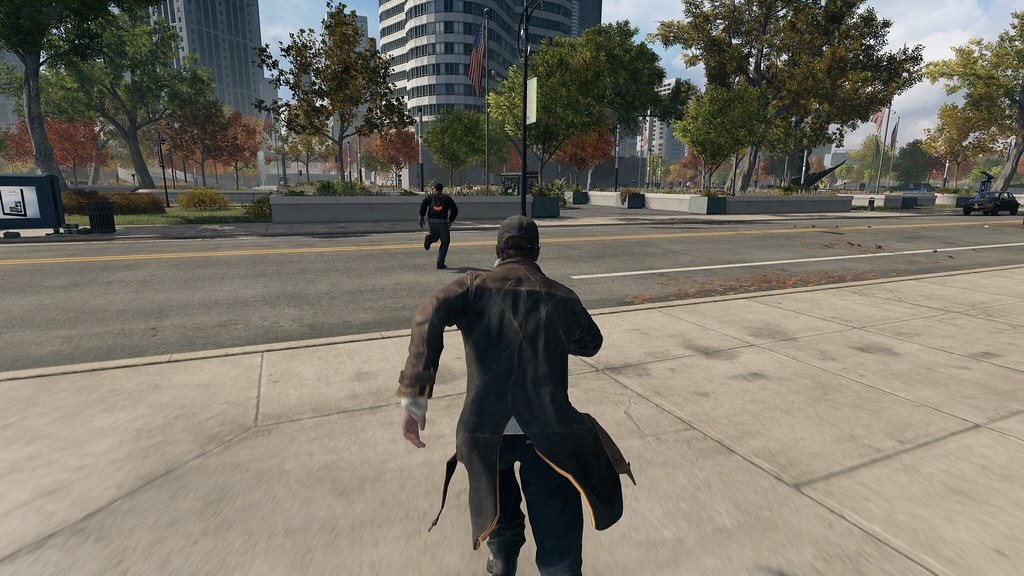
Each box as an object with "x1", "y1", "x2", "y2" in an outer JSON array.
[
  {"x1": 875, "y1": 139, "x2": 880, "y2": 157},
  {"x1": 869, "y1": 106, "x2": 886, "y2": 137},
  {"x1": 467, "y1": 20, "x2": 485, "y2": 100},
  {"x1": 890, "y1": 122, "x2": 899, "y2": 151}
]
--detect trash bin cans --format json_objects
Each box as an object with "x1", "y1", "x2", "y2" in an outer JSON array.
[
  {"x1": 85, "y1": 200, "x2": 116, "y2": 233},
  {"x1": 867, "y1": 197, "x2": 875, "y2": 211}
]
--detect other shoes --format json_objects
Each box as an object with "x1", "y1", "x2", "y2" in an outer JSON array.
[
  {"x1": 437, "y1": 265, "x2": 448, "y2": 269},
  {"x1": 424, "y1": 235, "x2": 432, "y2": 250}
]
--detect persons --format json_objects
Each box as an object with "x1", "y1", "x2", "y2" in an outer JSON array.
[
  {"x1": 417, "y1": 182, "x2": 458, "y2": 269},
  {"x1": 395, "y1": 216, "x2": 635, "y2": 576}
]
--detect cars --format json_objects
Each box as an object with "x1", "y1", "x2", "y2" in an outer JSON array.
[{"x1": 962, "y1": 191, "x2": 1021, "y2": 215}]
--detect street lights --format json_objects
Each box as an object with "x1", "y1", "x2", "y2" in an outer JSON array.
[
  {"x1": 157, "y1": 130, "x2": 171, "y2": 208},
  {"x1": 168, "y1": 151, "x2": 176, "y2": 190},
  {"x1": 875, "y1": 95, "x2": 897, "y2": 195},
  {"x1": 289, "y1": 141, "x2": 301, "y2": 185},
  {"x1": 271, "y1": 129, "x2": 280, "y2": 175}
]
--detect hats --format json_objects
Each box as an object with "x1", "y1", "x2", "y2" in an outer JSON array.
[
  {"x1": 432, "y1": 183, "x2": 443, "y2": 190},
  {"x1": 497, "y1": 215, "x2": 540, "y2": 250}
]
